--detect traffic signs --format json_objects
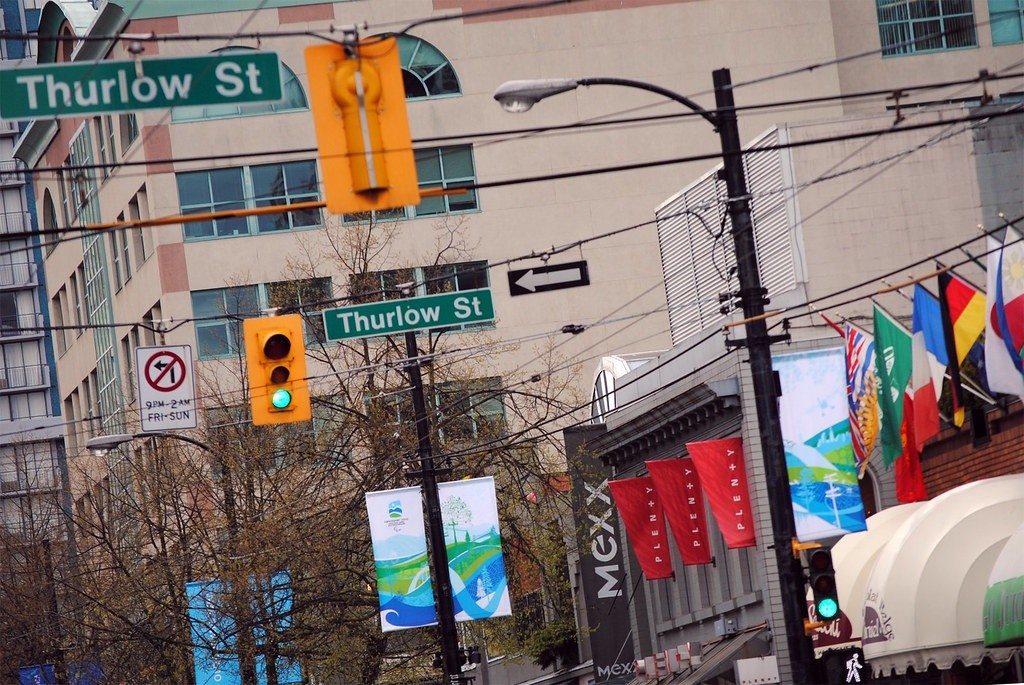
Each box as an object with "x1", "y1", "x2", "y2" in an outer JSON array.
[{"x1": 136, "y1": 345, "x2": 198, "y2": 431}]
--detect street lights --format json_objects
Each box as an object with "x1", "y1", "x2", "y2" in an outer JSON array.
[{"x1": 83, "y1": 430, "x2": 260, "y2": 685}]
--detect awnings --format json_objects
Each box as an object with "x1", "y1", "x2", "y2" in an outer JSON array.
[
  {"x1": 861, "y1": 472, "x2": 1024, "y2": 680},
  {"x1": 982, "y1": 520, "x2": 1024, "y2": 649},
  {"x1": 806, "y1": 500, "x2": 929, "y2": 659}
]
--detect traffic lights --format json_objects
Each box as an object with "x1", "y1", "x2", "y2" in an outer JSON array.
[
  {"x1": 492, "y1": 66, "x2": 817, "y2": 685},
  {"x1": 806, "y1": 545, "x2": 839, "y2": 622},
  {"x1": 243, "y1": 313, "x2": 311, "y2": 426}
]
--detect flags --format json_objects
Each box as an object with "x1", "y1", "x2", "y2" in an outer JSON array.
[{"x1": 844, "y1": 224, "x2": 1024, "y2": 503}]
